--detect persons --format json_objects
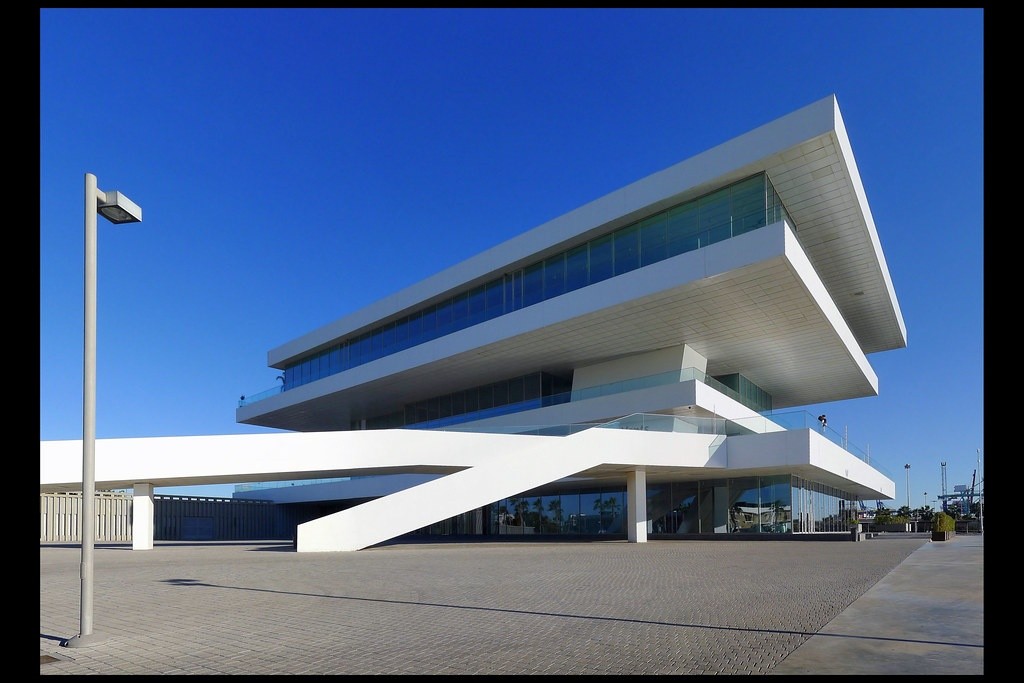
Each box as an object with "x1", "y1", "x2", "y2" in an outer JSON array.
[{"x1": 817, "y1": 414, "x2": 827, "y2": 431}]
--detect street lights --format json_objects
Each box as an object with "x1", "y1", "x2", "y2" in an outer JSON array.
[
  {"x1": 904, "y1": 463, "x2": 912, "y2": 510},
  {"x1": 63, "y1": 171, "x2": 148, "y2": 649},
  {"x1": 941, "y1": 461, "x2": 949, "y2": 512},
  {"x1": 977, "y1": 448, "x2": 984, "y2": 535},
  {"x1": 924, "y1": 491, "x2": 928, "y2": 508}
]
